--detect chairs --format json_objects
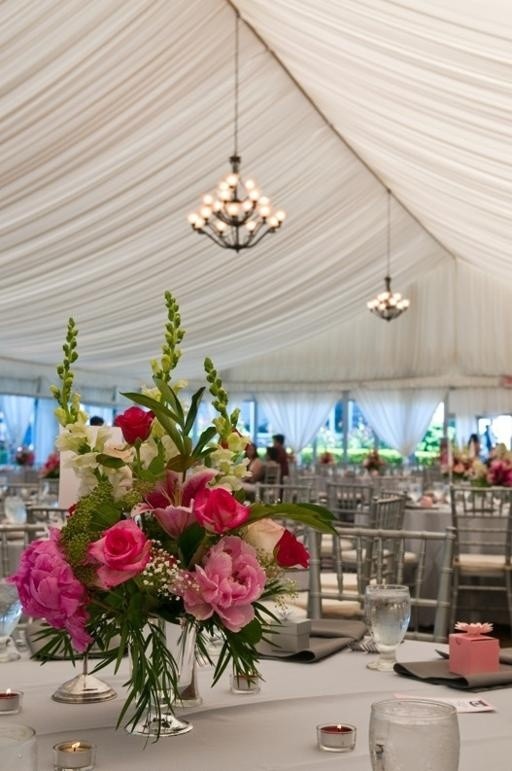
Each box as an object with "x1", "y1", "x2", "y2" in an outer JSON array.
[{"x1": 0, "y1": 479, "x2": 70, "y2": 578}]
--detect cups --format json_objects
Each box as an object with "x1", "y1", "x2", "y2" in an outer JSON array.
[
  {"x1": 0, "y1": 721, "x2": 39, "y2": 771},
  {"x1": 364, "y1": 695, "x2": 471, "y2": 770}
]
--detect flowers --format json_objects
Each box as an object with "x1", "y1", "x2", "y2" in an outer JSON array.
[
  {"x1": 17, "y1": 447, "x2": 34, "y2": 465},
  {"x1": 3, "y1": 292, "x2": 340, "y2": 751},
  {"x1": 37, "y1": 454, "x2": 60, "y2": 479}
]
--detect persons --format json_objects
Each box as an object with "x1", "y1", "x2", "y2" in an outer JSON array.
[
  {"x1": 89, "y1": 415, "x2": 105, "y2": 427},
  {"x1": 481, "y1": 424, "x2": 498, "y2": 452},
  {"x1": 243, "y1": 443, "x2": 264, "y2": 483},
  {"x1": 266, "y1": 446, "x2": 283, "y2": 482},
  {"x1": 271, "y1": 433, "x2": 288, "y2": 504}
]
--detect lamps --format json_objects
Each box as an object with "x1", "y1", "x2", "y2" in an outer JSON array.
[
  {"x1": 367, "y1": 190, "x2": 410, "y2": 321},
  {"x1": 188, "y1": 16, "x2": 286, "y2": 252}
]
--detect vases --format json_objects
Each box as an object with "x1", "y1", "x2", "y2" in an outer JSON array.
[{"x1": 124, "y1": 613, "x2": 192, "y2": 736}]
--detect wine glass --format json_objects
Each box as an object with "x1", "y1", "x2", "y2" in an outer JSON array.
[
  {"x1": 0, "y1": 576, "x2": 24, "y2": 663},
  {"x1": 408, "y1": 481, "x2": 447, "y2": 506},
  {"x1": 362, "y1": 583, "x2": 413, "y2": 672}
]
